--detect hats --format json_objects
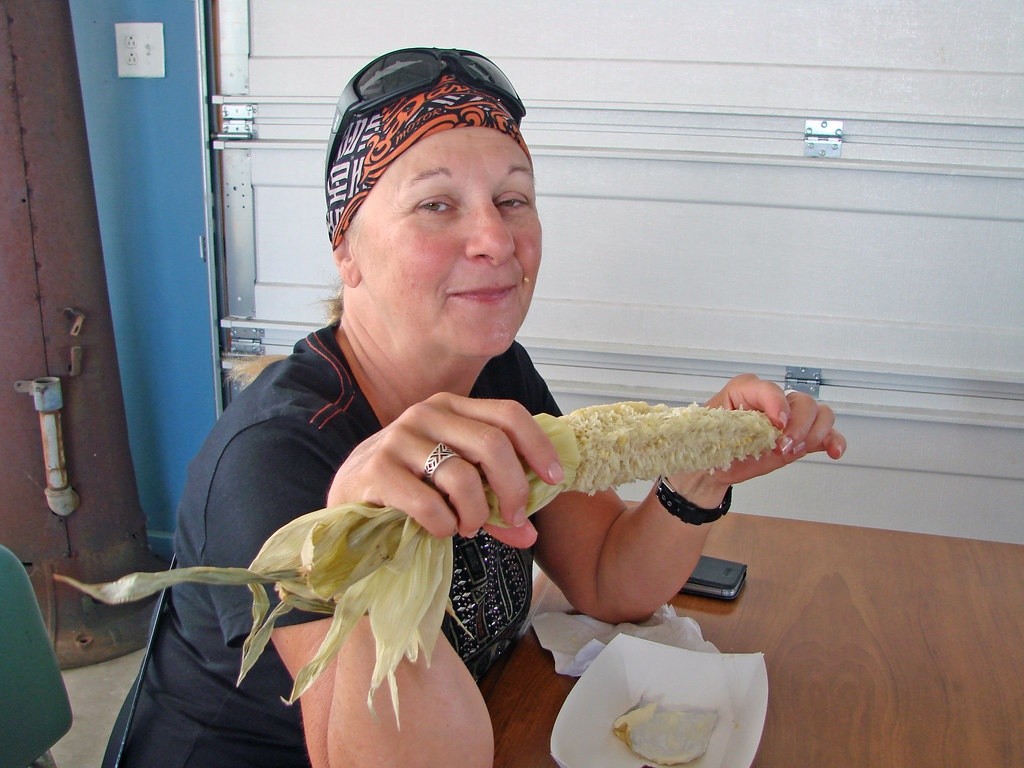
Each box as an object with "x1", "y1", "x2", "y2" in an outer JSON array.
[{"x1": 325, "y1": 61, "x2": 533, "y2": 251}]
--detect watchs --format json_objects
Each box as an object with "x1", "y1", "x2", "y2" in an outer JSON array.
[{"x1": 657, "y1": 473, "x2": 734, "y2": 525}]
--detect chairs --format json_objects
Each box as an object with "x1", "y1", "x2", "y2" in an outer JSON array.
[{"x1": 0, "y1": 543, "x2": 73, "y2": 768}]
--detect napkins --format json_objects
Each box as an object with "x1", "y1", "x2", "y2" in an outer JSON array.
[{"x1": 530, "y1": 602, "x2": 721, "y2": 676}]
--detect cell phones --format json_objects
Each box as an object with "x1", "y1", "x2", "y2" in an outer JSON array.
[{"x1": 680, "y1": 556, "x2": 747, "y2": 599}]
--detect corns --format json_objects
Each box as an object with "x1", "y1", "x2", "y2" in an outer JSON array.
[{"x1": 560, "y1": 400, "x2": 782, "y2": 495}]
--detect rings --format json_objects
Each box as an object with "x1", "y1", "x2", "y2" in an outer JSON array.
[{"x1": 422, "y1": 436, "x2": 458, "y2": 477}]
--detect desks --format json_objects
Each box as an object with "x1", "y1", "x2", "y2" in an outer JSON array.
[{"x1": 475, "y1": 499, "x2": 1024, "y2": 768}]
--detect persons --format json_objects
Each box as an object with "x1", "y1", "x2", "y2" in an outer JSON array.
[{"x1": 93, "y1": 49, "x2": 848, "y2": 768}]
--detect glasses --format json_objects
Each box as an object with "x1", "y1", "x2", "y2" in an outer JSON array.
[{"x1": 324, "y1": 47, "x2": 526, "y2": 188}]
